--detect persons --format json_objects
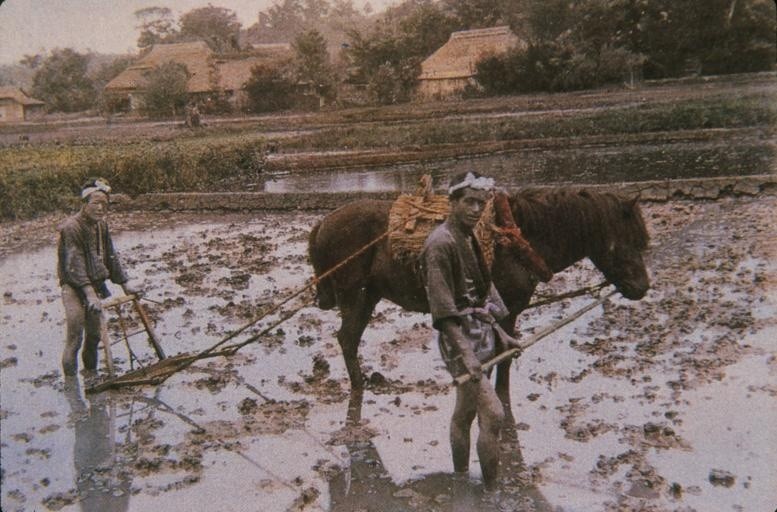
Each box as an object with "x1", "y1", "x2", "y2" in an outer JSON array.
[
  {"x1": 54, "y1": 178, "x2": 143, "y2": 379},
  {"x1": 61, "y1": 373, "x2": 131, "y2": 511},
  {"x1": 421, "y1": 170, "x2": 527, "y2": 504},
  {"x1": 190, "y1": 102, "x2": 202, "y2": 127}
]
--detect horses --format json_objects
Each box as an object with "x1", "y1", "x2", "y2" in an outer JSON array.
[{"x1": 309, "y1": 180, "x2": 651, "y2": 389}]
